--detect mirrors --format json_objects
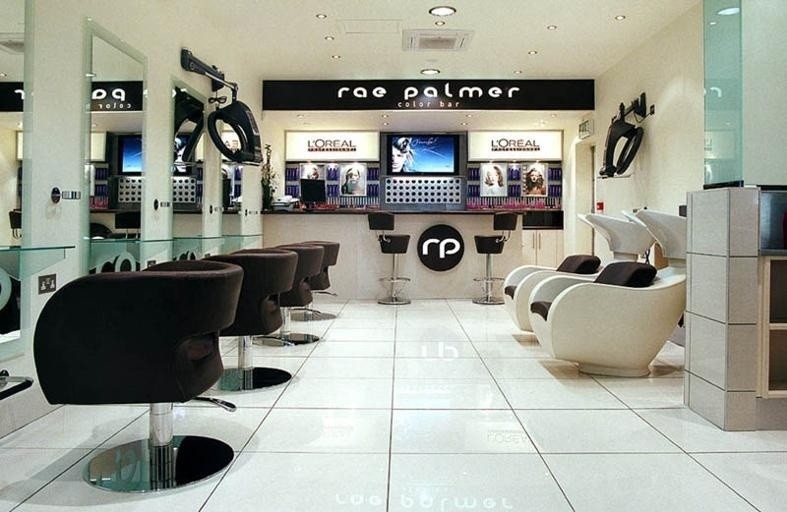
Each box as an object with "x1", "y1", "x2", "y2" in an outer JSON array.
[
  {"x1": 171, "y1": 72, "x2": 204, "y2": 262},
  {"x1": 220, "y1": 121, "x2": 243, "y2": 253},
  {"x1": 82, "y1": 14, "x2": 149, "y2": 280},
  {"x1": 0, "y1": 1, "x2": 32, "y2": 365}
]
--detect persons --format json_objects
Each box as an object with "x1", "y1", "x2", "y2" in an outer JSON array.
[
  {"x1": 392, "y1": 136, "x2": 416, "y2": 172},
  {"x1": 484, "y1": 166, "x2": 506, "y2": 195},
  {"x1": 526, "y1": 168, "x2": 546, "y2": 195},
  {"x1": 341, "y1": 167, "x2": 365, "y2": 195}
]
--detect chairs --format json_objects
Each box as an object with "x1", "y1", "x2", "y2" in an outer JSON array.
[
  {"x1": 472, "y1": 212, "x2": 517, "y2": 305},
  {"x1": 9, "y1": 211, "x2": 21, "y2": 239},
  {"x1": 107, "y1": 211, "x2": 140, "y2": 239},
  {"x1": 33, "y1": 261, "x2": 244, "y2": 494},
  {"x1": 501, "y1": 213, "x2": 656, "y2": 343},
  {"x1": 368, "y1": 212, "x2": 412, "y2": 305},
  {"x1": 290, "y1": 241, "x2": 339, "y2": 321},
  {"x1": 252, "y1": 243, "x2": 319, "y2": 346},
  {"x1": 201, "y1": 249, "x2": 298, "y2": 393},
  {"x1": 528, "y1": 207, "x2": 687, "y2": 377}
]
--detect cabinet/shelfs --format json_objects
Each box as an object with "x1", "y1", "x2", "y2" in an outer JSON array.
[
  {"x1": 467, "y1": 163, "x2": 562, "y2": 208},
  {"x1": 286, "y1": 164, "x2": 380, "y2": 209}
]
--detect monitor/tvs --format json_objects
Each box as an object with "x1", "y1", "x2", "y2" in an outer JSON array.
[
  {"x1": 387, "y1": 133, "x2": 458, "y2": 175},
  {"x1": 118, "y1": 134, "x2": 144, "y2": 175}
]
